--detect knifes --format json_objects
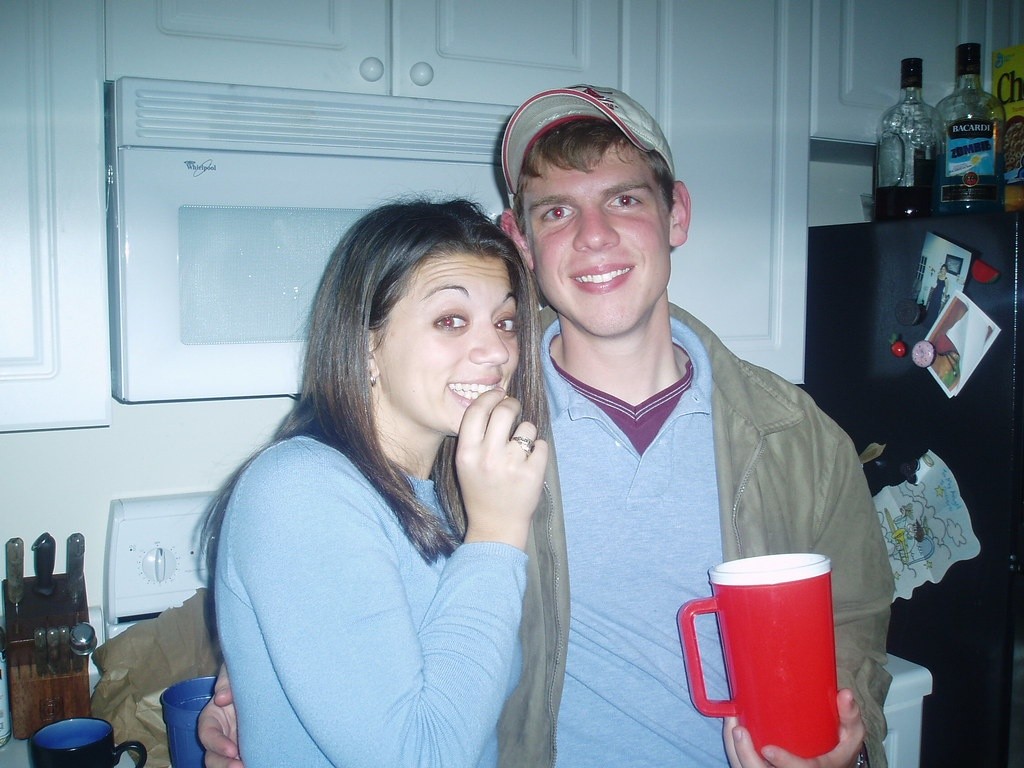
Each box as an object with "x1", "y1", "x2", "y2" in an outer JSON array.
[
  {"x1": 66, "y1": 532, "x2": 85, "y2": 606},
  {"x1": 44, "y1": 627, "x2": 59, "y2": 677},
  {"x1": 32, "y1": 533, "x2": 57, "y2": 603},
  {"x1": 33, "y1": 626, "x2": 47, "y2": 677},
  {"x1": 72, "y1": 651, "x2": 85, "y2": 674},
  {"x1": 5, "y1": 535, "x2": 25, "y2": 616},
  {"x1": 58, "y1": 625, "x2": 71, "y2": 675}
]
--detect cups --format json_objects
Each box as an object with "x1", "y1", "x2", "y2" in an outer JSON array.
[
  {"x1": 29, "y1": 718, "x2": 147, "y2": 768},
  {"x1": 676, "y1": 552, "x2": 842, "y2": 760},
  {"x1": 159, "y1": 676, "x2": 220, "y2": 768}
]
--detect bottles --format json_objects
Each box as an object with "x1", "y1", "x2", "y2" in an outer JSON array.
[
  {"x1": 933, "y1": 42, "x2": 1005, "y2": 214},
  {"x1": 0, "y1": 625, "x2": 12, "y2": 749},
  {"x1": 870, "y1": 58, "x2": 944, "y2": 221}
]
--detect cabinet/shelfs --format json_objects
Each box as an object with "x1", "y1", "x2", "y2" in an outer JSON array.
[
  {"x1": 0, "y1": 0, "x2": 1024, "y2": 433},
  {"x1": 796, "y1": 214, "x2": 1024, "y2": 768}
]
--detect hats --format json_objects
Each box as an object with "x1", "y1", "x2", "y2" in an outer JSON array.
[{"x1": 500, "y1": 83, "x2": 675, "y2": 209}]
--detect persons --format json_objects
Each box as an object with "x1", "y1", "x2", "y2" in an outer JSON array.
[
  {"x1": 918, "y1": 264, "x2": 949, "y2": 330},
  {"x1": 193, "y1": 197, "x2": 549, "y2": 768},
  {"x1": 196, "y1": 85, "x2": 896, "y2": 768}
]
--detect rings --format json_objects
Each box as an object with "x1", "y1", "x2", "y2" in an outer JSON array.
[{"x1": 510, "y1": 436, "x2": 535, "y2": 456}]
